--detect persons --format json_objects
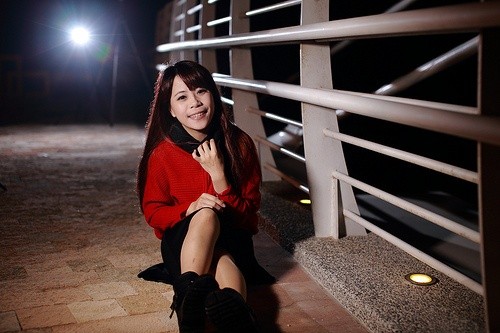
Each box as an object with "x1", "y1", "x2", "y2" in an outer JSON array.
[{"x1": 136, "y1": 59, "x2": 262, "y2": 333}]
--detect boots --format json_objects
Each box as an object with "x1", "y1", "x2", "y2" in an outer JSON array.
[{"x1": 171, "y1": 271, "x2": 251, "y2": 332}]
